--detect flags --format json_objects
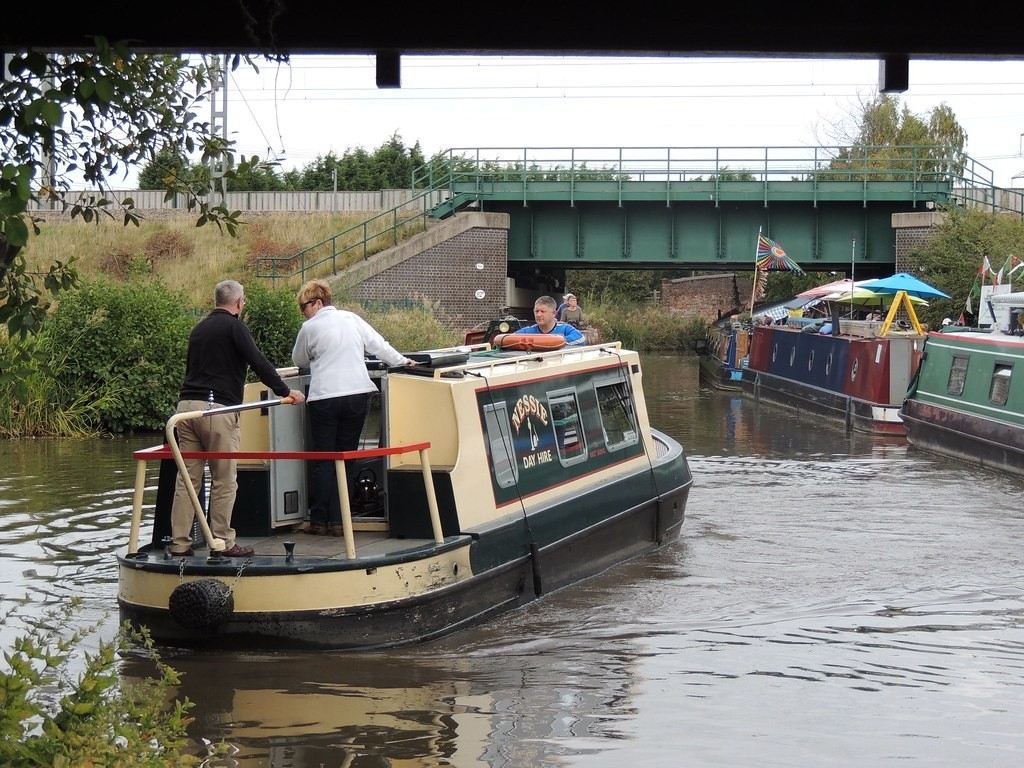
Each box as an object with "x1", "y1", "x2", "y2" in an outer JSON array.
[
  {"x1": 958, "y1": 255, "x2": 1024, "y2": 327},
  {"x1": 756, "y1": 236, "x2": 806, "y2": 275}
]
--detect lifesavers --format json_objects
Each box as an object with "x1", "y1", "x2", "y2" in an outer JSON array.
[
  {"x1": 493, "y1": 334, "x2": 566, "y2": 351},
  {"x1": 897, "y1": 320, "x2": 908, "y2": 328},
  {"x1": 731, "y1": 315, "x2": 739, "y2": 319}
]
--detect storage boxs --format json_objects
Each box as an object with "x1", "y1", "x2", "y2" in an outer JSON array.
[{"x1": 839, "y1": 320, "x2": 884, "y2": 337}]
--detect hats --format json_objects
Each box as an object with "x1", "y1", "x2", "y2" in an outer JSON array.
[{"x1": 562, "y1": 293, "x2": 577, "y2": 301}]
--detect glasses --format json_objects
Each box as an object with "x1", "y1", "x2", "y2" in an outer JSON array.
[{"x1": 299, "y1": 298, "x2": 317, "y2": 312}]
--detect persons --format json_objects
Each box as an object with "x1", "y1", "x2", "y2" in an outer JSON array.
[
  {"x1": 560, "y1": 295, "x2": 585, "y2": 329},
  {"x1": 170, "y1": 280, "x2": 305, "y2": 557},
  {"x1": 555, "y1": 294, "x2": 573, "y2": 321},
  {"x1": 292, "y1": 279, "x2": 418, "y2": 536},
  {"x1": 513, "y1": 295, "x2": 587, "y2": 459}
]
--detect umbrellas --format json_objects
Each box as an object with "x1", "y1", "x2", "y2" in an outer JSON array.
[{"x1": 783, "y1": 273, "x2": 951, "y2": 321}]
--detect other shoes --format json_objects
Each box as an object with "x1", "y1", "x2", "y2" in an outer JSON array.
[
  {"x1": 303, "y1": 521, "x2": 343, "y2": 537},
  {"x1": 222, "y1": 544, "x2": 255, "y2": 557},
  {"x1": 171, "y1": 549, "x2": 194, "y2": 556}
]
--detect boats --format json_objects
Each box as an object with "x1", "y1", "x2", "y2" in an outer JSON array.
[
  {"x1": 112, "y1": 333, "x2": 692, "y2": 655},
  {"x1": 898, "y1": 293, "x2": 1024, "y2": 454},
  {"x1": 736, "y1": 295, "x2": 928, "y2": 429},
  {"x1": 697, "y1": 306, "x2": 754, "y2": 391}
]
